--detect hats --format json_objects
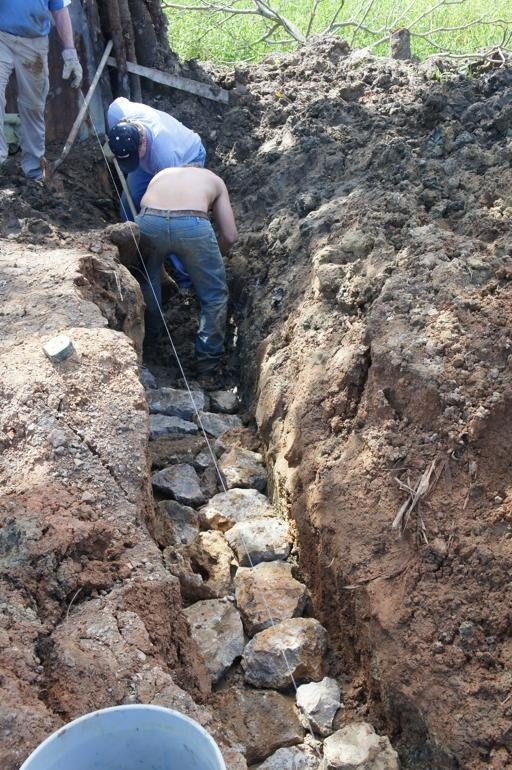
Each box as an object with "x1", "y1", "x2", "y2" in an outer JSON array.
[{"x1": 108, "y1": 122, "x2": 139, "y2": 173}]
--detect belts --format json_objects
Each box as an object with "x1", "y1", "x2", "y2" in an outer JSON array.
[{"x1": 141, "y1": 206, "x2": 209, "y2": 220}]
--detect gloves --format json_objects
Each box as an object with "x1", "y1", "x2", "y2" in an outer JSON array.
[{"x1": 61, "y1": 48, "x2": 83, "y2": 89}]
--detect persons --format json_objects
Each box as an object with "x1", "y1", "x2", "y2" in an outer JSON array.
[
  {"x1": 102, "y1": 96, "x2": 207, "y2": 310},
  {"x1": 130, "y1": 165, "x2": 241, "y2": 390},
  {"x1": 1, "y1": 0, "x2": 84, "y2": 189}
]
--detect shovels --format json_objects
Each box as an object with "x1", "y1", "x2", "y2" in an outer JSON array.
[
  {"x1": 41, "y1": 39, "x2": 114, "y2": 197},
  {"x1": 104, "y1": 132, "x2": 182, "y2": 306}
]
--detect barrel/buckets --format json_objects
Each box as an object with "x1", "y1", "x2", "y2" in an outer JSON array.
[{"x1": 17, "y1": 703, "x2": 225, "y2": 770}]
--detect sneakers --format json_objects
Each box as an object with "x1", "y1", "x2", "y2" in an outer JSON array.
[{"x1": 27, "y1": 169, "x2": 43, "y2": 181}]
